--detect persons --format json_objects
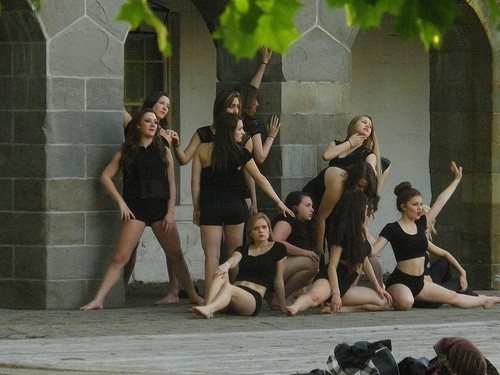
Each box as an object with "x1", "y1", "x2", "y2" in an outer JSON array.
[{"x1": 78, "y1": 90, "x2": 500, "y2": 318}]
